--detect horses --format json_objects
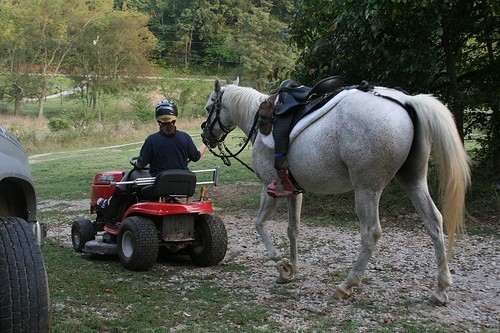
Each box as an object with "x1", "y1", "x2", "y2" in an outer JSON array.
[{"x1": 200, "y1": 74, "x2": 472, "y2": 306}]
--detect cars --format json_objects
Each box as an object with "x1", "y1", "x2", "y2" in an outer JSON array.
[{"x1": 0, "y1": 124, "x2": 50, "y2": 333}]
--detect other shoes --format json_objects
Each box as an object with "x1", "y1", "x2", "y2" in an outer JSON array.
[{"x1": 97, "y1": 196, "x2": 112, "y2": 209}]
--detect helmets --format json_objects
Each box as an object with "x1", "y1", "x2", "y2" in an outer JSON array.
[{"x1": 155, "y1": 100, "x2": 178, "y2": 124}]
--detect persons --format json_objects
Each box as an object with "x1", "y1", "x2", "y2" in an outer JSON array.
[{"x1": 97, "y1": 101, "x2": 210, "y2": 220}]
